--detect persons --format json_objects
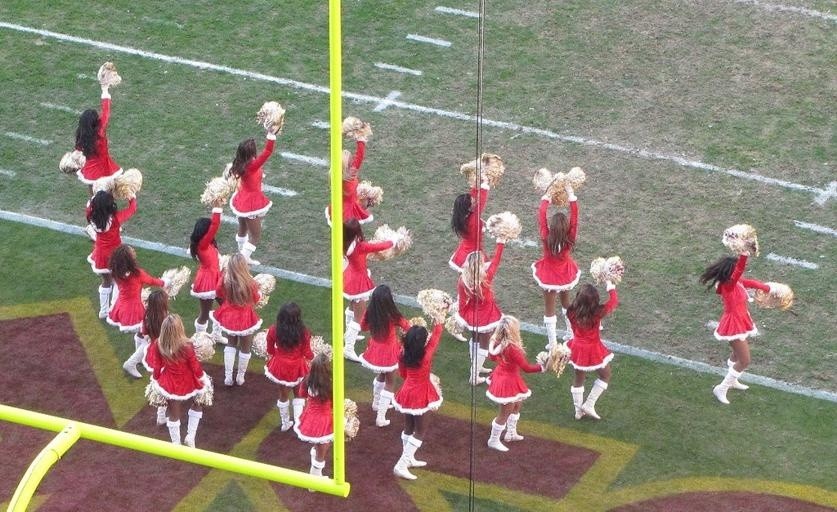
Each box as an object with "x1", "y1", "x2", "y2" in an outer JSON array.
[
  {"x1": 321, "y1": 140, "x2": 376, "y2": 233},
  {"x1": 485, "y1": 316, "x2": 541, "y2": 451},
  {"x1": 564, "y1": 277, "x2": 618, "y2": 424},
  {"x1": 531, "y1": 178, "x2": 581, "y2": 352},
  {"x1": 105, "y1": 245, "x2": 213, "y2": 448},
  {"x1": 694, "y1": 248, "x2": 772, "y2": 405},
  {"x1": 222, "y1": 125, "x2": 280, "y2": 267},
  {"x1": 189, "y1": 198, "x2": 223, "y2": 334},
  {"x1": 357, "y1": 282, "x2": 445, "y2": 481},
  {"x1": 454, "y1": 237, "x2": 506, "y2": 386},
  {"x1": 447, "y1": 175, "x2": 491, "y2": 275},
  {"x1": 213, "y1": 254, "x2": 260, "y2": 387},
  {"x1": 342, "y1": 220, "x2": 396, "y2": 363},
  {"x1": 261, "y1": 297, "x2": 334, "y2": 495},
  {"x1": 65, "y1": 81, "x2": 123, "y2": 243}
]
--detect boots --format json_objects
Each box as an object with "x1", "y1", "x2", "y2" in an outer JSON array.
[
  {"x1": 712, "y1": 358, "x2": 749, "y2": 404},
  {"x1": 84, "y1": 222, "x2": 260, "y2": 448}
]
